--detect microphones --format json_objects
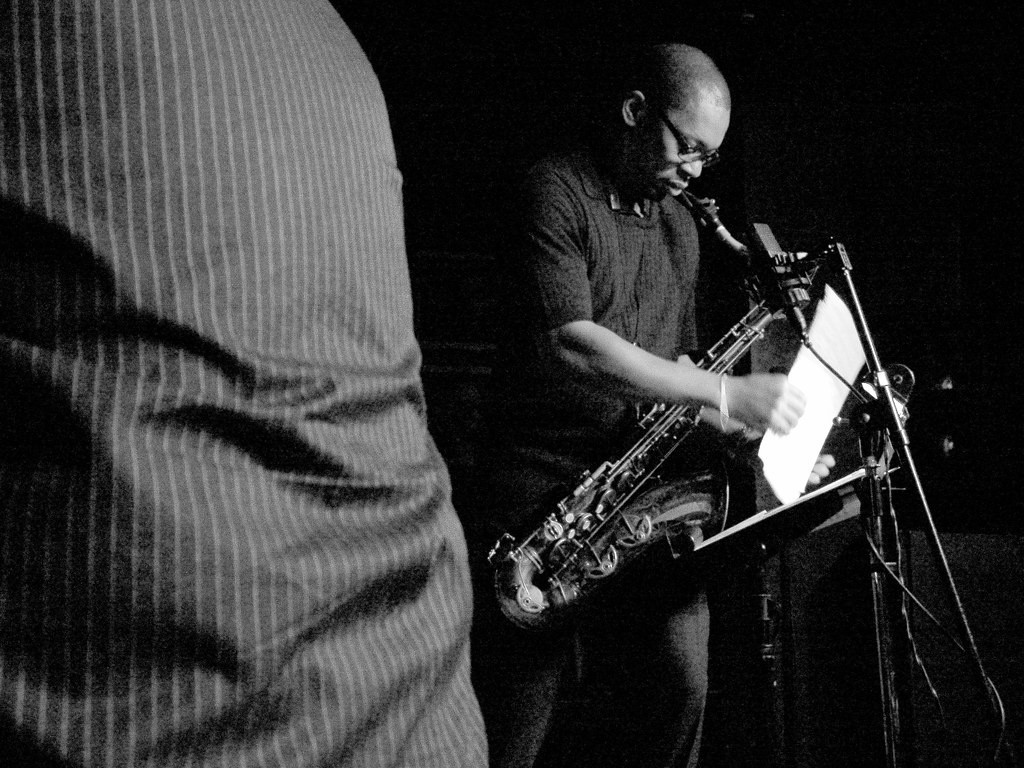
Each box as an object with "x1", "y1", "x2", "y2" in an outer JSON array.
[{"x1": 742, "y1": 223, "x2": 812, "y2": 346}]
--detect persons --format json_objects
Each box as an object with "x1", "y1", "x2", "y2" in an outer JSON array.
[
  {"x1": 485, "y1": 46, "x2": 837, "y2": 768},
  {"x1": 0, "y1": 1, "x2": 484, "y2": 768}
]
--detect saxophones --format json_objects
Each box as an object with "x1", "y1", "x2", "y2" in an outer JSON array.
[{"x1": 483, "y1": 188, "x2": 823, "y2": 632}]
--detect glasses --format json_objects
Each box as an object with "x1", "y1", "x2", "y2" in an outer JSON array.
[{"x1": 642, "y1": 98, "x2": 721, "y2": 168}]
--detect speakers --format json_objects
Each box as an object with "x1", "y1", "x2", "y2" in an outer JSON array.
[{"x1": 779, "y1": 529, "x2": 1024, "y2": 768}]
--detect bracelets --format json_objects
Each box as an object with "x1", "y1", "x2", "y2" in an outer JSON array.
[{"x1": 718, "y1": 373, "x2": 728, "y2": 420}]
